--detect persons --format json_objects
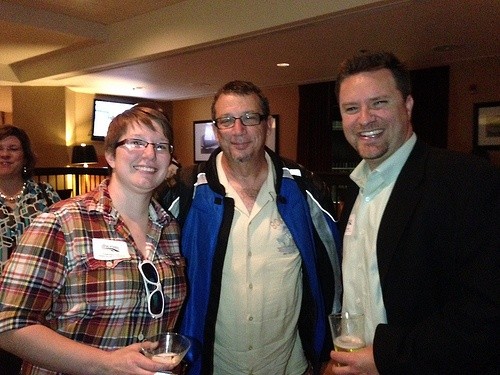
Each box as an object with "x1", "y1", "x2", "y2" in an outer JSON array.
[
  {"x1": 161, "y1": 79, "x2": 344, "y2": 375},
  {"x1": 0, "y1": 124, "x2": 63, "y2": 375},
  {"x1": 0, "y1": 106, "x2": 187, "y2": 375},
  {"x1": 329, "y1": 48, "x2": 500, "y2": 375}
]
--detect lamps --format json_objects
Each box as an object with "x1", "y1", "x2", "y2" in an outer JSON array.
[{"x1": 73, "y1": 143, "x2": 98, "y2": 169}]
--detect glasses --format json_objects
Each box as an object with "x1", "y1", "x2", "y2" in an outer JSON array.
[
  {"x1": 114, "y1": 137, "x2": 174, "y2": 154},
  {"x1": 212, "y1": 112, "x2": 265, "y2": 129},
  {"x1": 138, "y1": 258, "x2": 165, "y2": 319}
]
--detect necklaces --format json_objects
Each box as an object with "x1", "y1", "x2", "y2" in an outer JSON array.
[{"x1": 0, "y1": 181, "x2": 27, "y2": 201}]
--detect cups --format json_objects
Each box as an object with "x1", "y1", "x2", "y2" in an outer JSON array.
[
  {"x1": 140, "y1": 332, "x2": 191, "y2": 370},
  {"x1": 329, "y1": 311, "x2": 367, "y2": 368}
]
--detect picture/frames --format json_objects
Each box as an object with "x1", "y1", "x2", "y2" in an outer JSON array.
[
  {"x1": 192, "y1": 115, "x2": 280, "y2": 165},
  {"x1": 472, "y1": 101, "x2": 500, "y2": 151}
]
